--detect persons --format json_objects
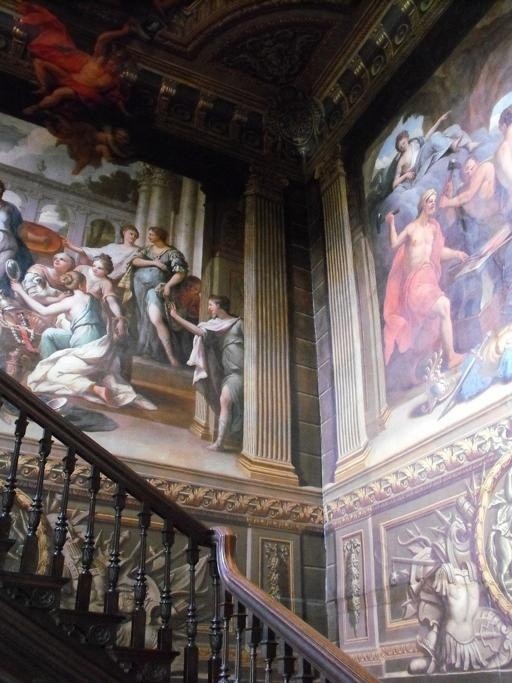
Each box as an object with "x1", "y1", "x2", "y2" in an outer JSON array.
[
  {"x1": 0, "y1": 181, "x2": 250, "y2": 453},
  {"x1": 46, "y1": 107, "x2": 135, "y2": 170},
  {"x1": 19, "y1": 22, "x2": 152, "y2": 121},
  {"x1": 379, "y1": 104, "x2": 512, "y2": 405}
]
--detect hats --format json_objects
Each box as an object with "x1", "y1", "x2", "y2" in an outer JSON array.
[
  {"x1": 19, "y1": 218, "x2": 62, "y2": 253},
  {"x1": 2, "y1": 189, "x2": 25, "y2": 225}
]
